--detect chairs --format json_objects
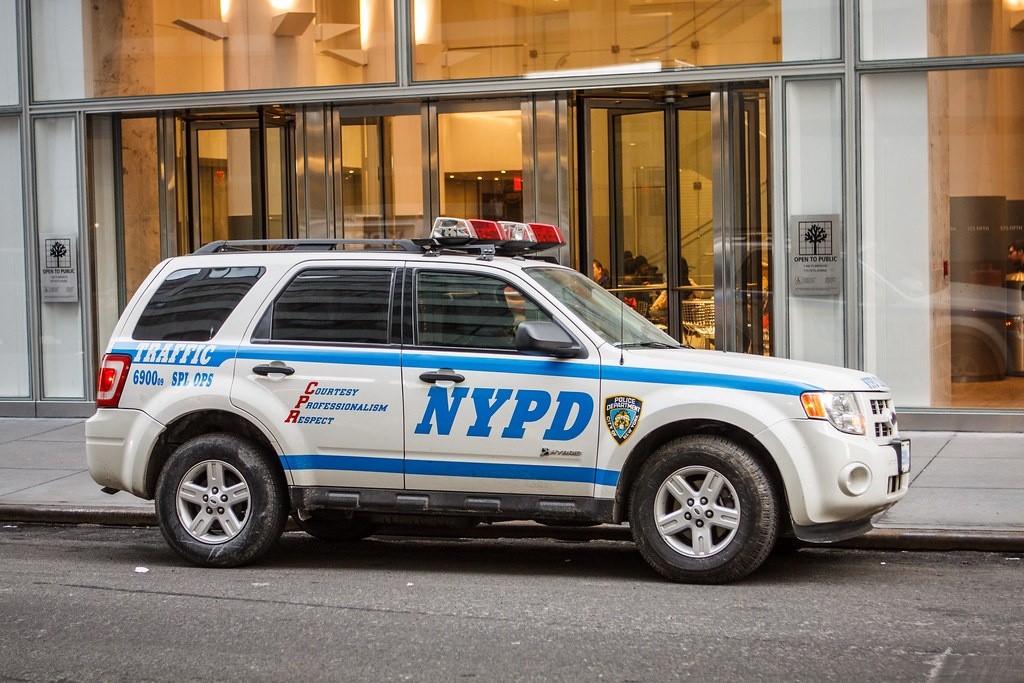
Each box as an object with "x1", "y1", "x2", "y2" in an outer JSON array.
[
  {"x1": 622, "y1": 272, "x2": 716, "y2": 350},
  {"x1": 443, "y1": 296, "x2": 481, "y2": 346}
]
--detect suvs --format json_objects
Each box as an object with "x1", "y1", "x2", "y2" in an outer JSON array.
[
  {"x1": 84, "y1": 216, "x2": 912, "y2": 586},
  {"x1": 711, "y1": 231, "x2": 1024, "y2": 382}
]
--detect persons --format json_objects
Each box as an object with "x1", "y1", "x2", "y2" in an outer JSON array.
[
  {"x1": 650, "y1": 255, "x2": 704, "y2": 313},
  {"x1": 623, "y1": 250, "x2": 664, "y2": 304},
  {"x1": 1008, "y1": 240, "x2": 1024, "y2": 325},
  {"x1": 592, "y1": 260, "x2": 611, "y2": 289}
]
